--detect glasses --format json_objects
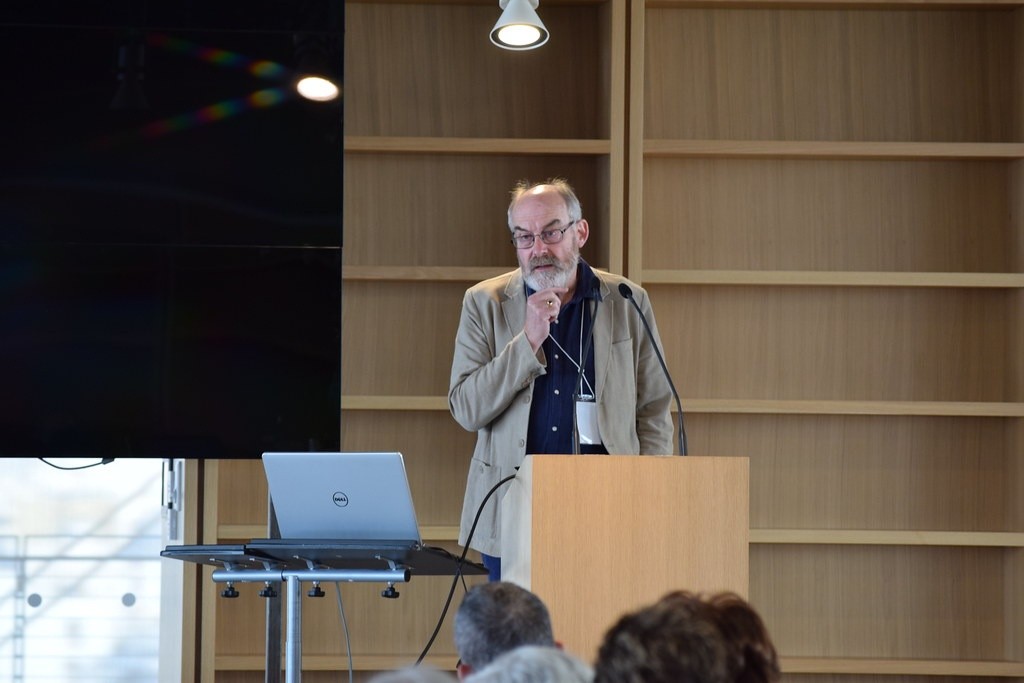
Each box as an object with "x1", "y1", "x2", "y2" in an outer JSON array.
[{"x1": 510, "y1": 221, "x2": 574, "y2": 249}]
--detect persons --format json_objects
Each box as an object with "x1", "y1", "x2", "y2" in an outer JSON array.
[
  {"x1": 463, "y1": 645, "x2": 595, "y2": 683},
  {"x1": 590, "y1": 588, "x2": 782, "y2": 683},
  {"x1": 454, "y1": 581, "x2": 565, "y2": 679},
  {"x1": 449, "y1": 178, "x2": 675, "y2": 580}
]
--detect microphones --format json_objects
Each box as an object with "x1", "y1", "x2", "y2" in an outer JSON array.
[
  {"x1": 618, "y1": 282, "x2": 692, "y2": 457},
  {"x1": 572, "y1": 276, "x2": 600, "y2": 455}
]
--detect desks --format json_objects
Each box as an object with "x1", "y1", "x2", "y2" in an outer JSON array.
[{"x1": 160, "y1": 539, "x2": 490, "y2": 683}]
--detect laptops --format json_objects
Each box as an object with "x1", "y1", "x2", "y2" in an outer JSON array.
[{"x1": 262, "y1": 451, "x2": 433, "y2": 549}]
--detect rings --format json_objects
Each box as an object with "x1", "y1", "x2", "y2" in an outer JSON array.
[{"x1": 546, "y1": 300, "x2": 553, "y2": 307}]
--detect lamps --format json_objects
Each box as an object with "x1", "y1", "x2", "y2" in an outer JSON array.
[{"x1": 490, "y1": 0, "x2": 550, "y2": 51}]
